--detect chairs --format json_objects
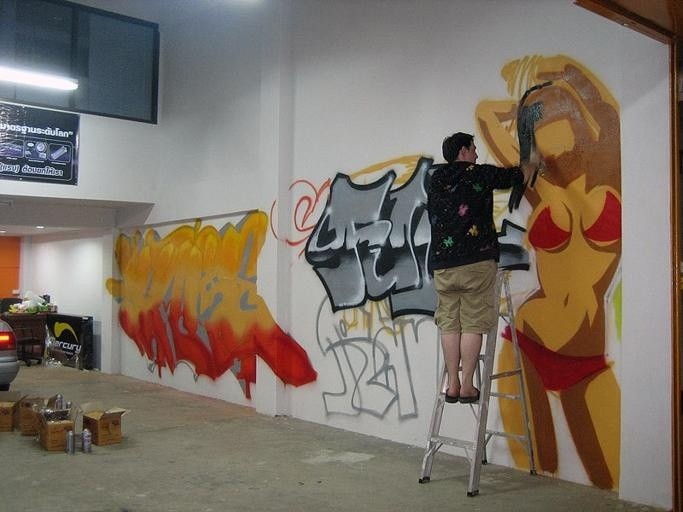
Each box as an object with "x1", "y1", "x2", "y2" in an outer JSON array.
[{"x1": 1, "y1": 311, "x2": 45, "y2": 366}]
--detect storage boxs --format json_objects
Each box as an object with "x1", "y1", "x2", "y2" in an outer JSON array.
[{"x1": 0, "y1": 393, "x2": 130, "y2": 452}]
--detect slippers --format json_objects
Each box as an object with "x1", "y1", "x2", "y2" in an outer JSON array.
[
  {"x1": 459, "y1": 387, "x2": 480, "y2": 402},
  {"x1": 446, "y1": 388, "x2": 459, "y2": 403}
]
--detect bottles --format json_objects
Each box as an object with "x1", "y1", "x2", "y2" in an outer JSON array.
[
  {"x1": 65, "y1": 430, "x2": 75, "y2": 455},
  {"x1": 55, "y1": 392, "x2": 63, "y2": 410},
  {"x1": 81, "y1": 428, "x2": 91, "y2": 453},
  {"x1": 66, "y1": 400, "x2": 72, "y2": 409}
]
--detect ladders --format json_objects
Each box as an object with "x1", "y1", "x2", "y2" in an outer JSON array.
[{"x1": 417, "y1": 265, "x2": 537, "y2": 498}]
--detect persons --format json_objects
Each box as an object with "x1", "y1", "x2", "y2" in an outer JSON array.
[
  {"x1": 475, "y1": 56, "x2": 620, "y2": 496},
  {"x1": 423, "y1": 133, "x2": 541, "y2": 404}
]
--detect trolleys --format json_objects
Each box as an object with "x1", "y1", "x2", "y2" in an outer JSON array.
[{"x1": 14, "y1": 328, "x2": 43, "y2": 367}]
